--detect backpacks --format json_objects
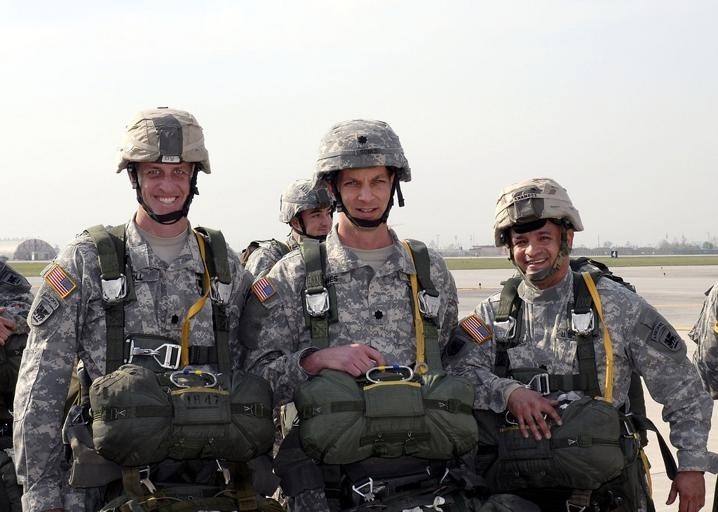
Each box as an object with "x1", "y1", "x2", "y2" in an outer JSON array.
[
  {"x1": 488, "y1": 271, "x2": 637, "y2": 492},
  {"x1": 298, "y1": 238, "x2": 480, "y2": 463}
]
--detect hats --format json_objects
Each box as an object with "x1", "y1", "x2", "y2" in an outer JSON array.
[{"x1": 313, "y1": 120, "x2": 412, "y2": 189}]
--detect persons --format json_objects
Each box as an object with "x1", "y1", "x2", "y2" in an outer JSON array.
[
  {"x1": 687, "y1": 278, "x2": 717, "y2": 401},
  {"x1": 449, "y1": 178, "x2": 718, "y2": 511},
  {"x1": 10, "y1": 105, "x2": 283, "y2": 510},
  {"x1": 1, "y1": 261, "x2": 34, "y2": 511},
  {"x1": 236, "y1": 119, "x2": 457, "y2": 508},
  {"x1": 241, "y1": 176, "x2": 335, "y2": 279}
]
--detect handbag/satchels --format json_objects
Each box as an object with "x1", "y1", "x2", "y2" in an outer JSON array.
[
  {"x1": 103, "y1": 485, "x2": 282, "y2": 512},
  {"x1": 350, "y1": 468, "x2": 471, "y2": 512},
  {"x1": 89, "y1": 228, "x2": 274, "y2": 461}
]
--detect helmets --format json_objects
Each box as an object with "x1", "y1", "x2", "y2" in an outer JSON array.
[
  {"x1": 494, "y1": 177, "x2": 583, "y2": 248},
  {"x1": 278, "y1": 178, "x2": 335, "y2": 223},
  {"x1": 116, "y1": 105, "x2": 211, "y2": 176}
]
